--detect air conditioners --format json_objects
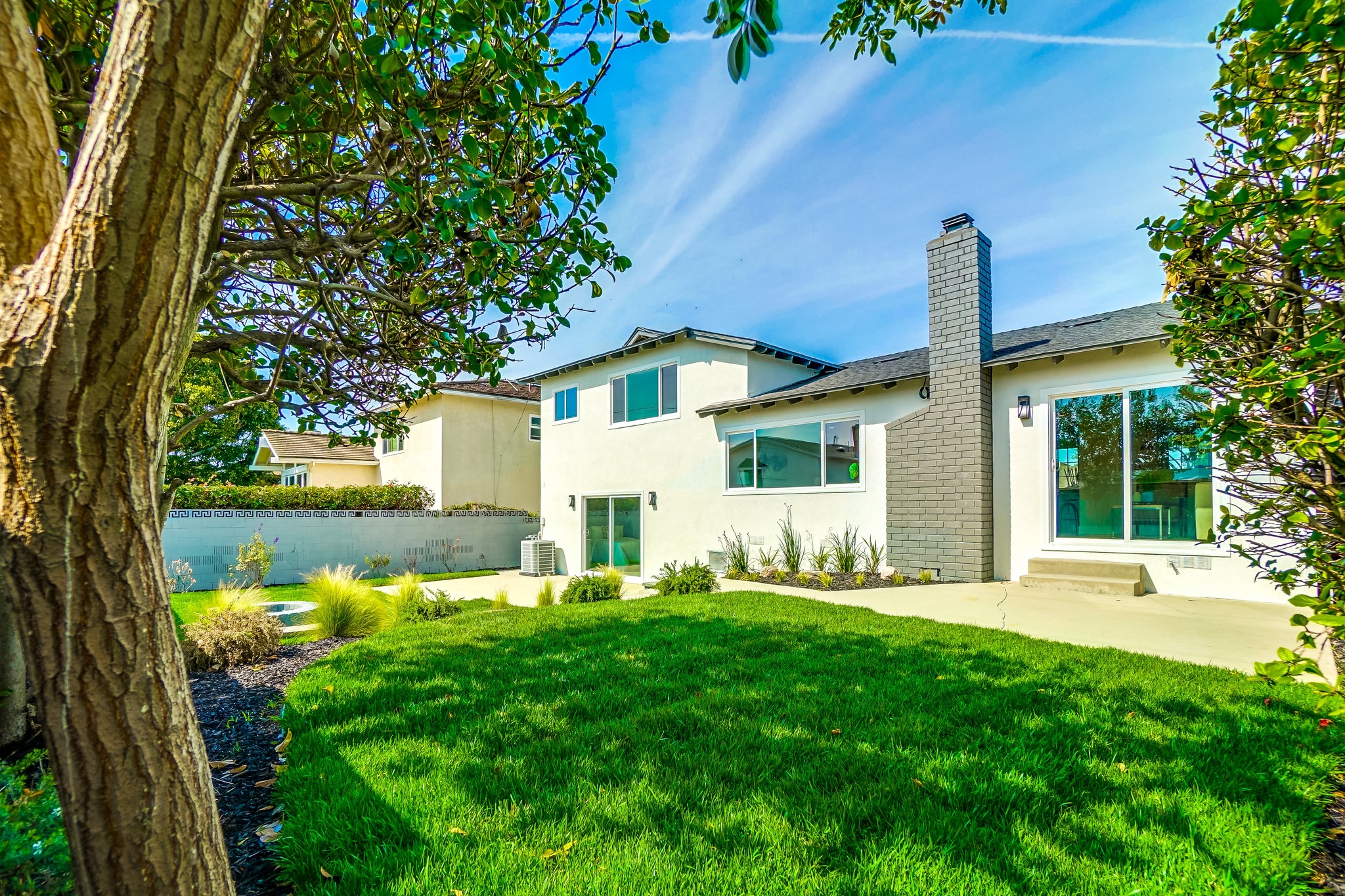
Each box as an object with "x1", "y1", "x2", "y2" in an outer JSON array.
[{"x1": 520, "y1": 540, "x2": 556, "y2": 577}]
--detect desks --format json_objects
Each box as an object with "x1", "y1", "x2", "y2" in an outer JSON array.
[
  {"x1": 587, "y1": 539, "x2": 591, "y2": 570},
  {"x1": 1112, "y1": 504, "x2": 1171, "y2": 540}
]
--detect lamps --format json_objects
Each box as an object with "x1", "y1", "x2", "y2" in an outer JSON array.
[
  {"x1": 1140, "y1": 423, "x2": 1153, "y2": 450},
  {"x1": 648, "y1": 492, "x2": 656, "y2": 505},
  {"x1": 569, "y1": 495, "x2": 575, "y2": 507},
  {"x1": 1018, "y1": 395, "x2": 1030, "y2": 419}
]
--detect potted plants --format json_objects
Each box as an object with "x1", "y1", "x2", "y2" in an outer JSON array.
[{"x1": 1137, "y1": 471, "x2": 1162, "y2": 502}]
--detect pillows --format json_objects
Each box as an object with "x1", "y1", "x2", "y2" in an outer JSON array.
[{"x1": 590, "y1": 525, "x2": 624, "y2": 540}]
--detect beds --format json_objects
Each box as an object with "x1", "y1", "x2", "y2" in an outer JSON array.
[{"x1": 588, "y1": 527, "x2": 640, "y2": 564}]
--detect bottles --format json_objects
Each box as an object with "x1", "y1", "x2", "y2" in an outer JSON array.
[{"x1": 538, "y1": 531, "x2": 542, "y2": 540}]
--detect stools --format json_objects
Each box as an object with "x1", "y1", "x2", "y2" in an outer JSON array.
[{"x1": 1058, "y1": 499, "x2": 1079, "y2": 534}]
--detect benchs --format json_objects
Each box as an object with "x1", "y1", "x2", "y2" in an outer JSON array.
[{"x1": 1132, "y1": 482, "x2": 1188, "y2": 538}]
